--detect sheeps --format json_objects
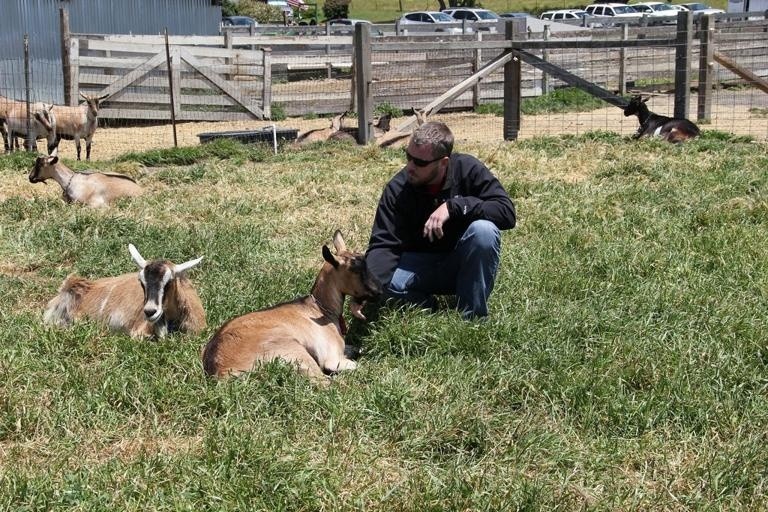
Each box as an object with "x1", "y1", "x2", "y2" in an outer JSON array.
[
  {"x1": 199, "y1": 228, "x2": 385, "y2": 395},
  {"x1": 624, "y1": 93, "x2": 701, "y2": 146},
  {"x1": 374, "y1": 107, "x2": 433, "y2": 150},
  {"x1": 0, "y1": 90, "x2": 110, "y2": 165},
  {"x1": 295, "y1": 110, "x2": 347, "y2": 146},
  {"x1": 28, "y1": 146, "x2": 143, "y2": 210},
  {"x1": 326, "y1": 112, "x2": 392, "y2": 147},
  {"x1": 42, "y1": 243, "x2": 208, "y2": 344}
]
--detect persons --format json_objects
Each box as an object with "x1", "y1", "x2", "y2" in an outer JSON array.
[{"x1": 349, "y1": 122, "x2": 516, "y2": 327}]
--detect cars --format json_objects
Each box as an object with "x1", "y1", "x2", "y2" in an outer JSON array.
[
  {"x1": 222, "y1": 15, "x2": 257, "y2": 25},
  {"x1": 267, "y1": 0, "x2": 293, "y2": 23},
  {"x1": 329, "y1": 19, "x2": 373, "y2": 26}
]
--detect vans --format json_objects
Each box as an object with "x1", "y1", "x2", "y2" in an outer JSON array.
[
  {"x1": 399, "y1": 9, "x2": 531, "y2": 32},
  {"x1": 540, "y1": 2, "x2": 726, "y2": 28}
]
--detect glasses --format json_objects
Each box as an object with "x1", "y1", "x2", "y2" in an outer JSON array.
[{"x1": 406, "y1": 151, "x2": 445, "y2": 167}]
